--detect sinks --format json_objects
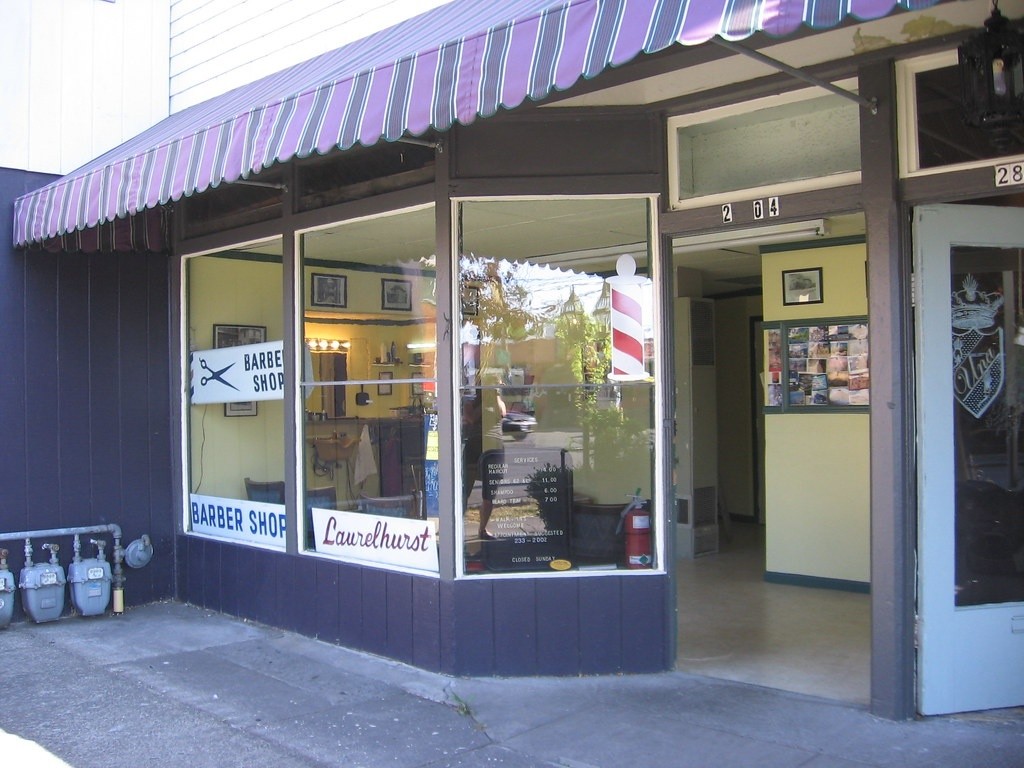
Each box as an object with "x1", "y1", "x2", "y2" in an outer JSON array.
[{"x1": 313, "y1": 439, "x2": 357, "y2": 460}]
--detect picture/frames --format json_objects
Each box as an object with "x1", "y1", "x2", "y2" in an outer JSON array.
[
  {"x1": 377, "y1": 372, "x2": 393, "y2": 395},
  {"x1": 213, "y1": 324, "x2": 267, "y2": 350},
  {"x1": 311, "y1": 273, "x2": 348, "y2": 308},
  {"x1": 224, "y1": 401, "x2": 260, "y2": 416},
  {"x1": 782, "y1": 267, "x2": 824, "y2": 306},
  {"x1": 380, "y1": 279, "x2": 413, "y2": 311},
  {"x1": 412, "y1": 372, "x2": 424, "y2": 396},
  {"x1": 462, "y1": 285, "x2": 480, "y2": 316}
]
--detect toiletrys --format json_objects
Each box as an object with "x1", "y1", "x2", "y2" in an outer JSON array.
[{"x1": 304, "y1": 408, "x2": 328, "y2": 422}]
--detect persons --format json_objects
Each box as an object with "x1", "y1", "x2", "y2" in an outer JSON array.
[{"x1": 461, "y1": 377, "x2": 506, "y2": 539}]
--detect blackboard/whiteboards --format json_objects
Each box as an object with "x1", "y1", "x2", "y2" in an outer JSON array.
[{"x1": 479, "y1": 448, "x2": 574, "y2": 573}]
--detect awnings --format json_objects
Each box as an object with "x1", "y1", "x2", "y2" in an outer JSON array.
[{"x1": 12, "y1": 0, "x2": 939, "y2": 252}]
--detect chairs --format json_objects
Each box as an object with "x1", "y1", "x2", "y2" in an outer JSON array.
[{"x1": 243, "y1": 477, "x2": 414, "y2": 547}]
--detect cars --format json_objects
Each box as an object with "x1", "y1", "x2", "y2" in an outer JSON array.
[{"x1": 460, "y1": 395, "x2": 538, "y2": 441}]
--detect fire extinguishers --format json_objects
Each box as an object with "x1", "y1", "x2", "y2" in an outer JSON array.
[{"x1": 624, "y1": 494, "x2": 652, "y2": 569}]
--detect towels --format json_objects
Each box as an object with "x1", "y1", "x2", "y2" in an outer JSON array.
[{"x1": 354, "y1": 424, "x2": 377, "y2": 486}]
